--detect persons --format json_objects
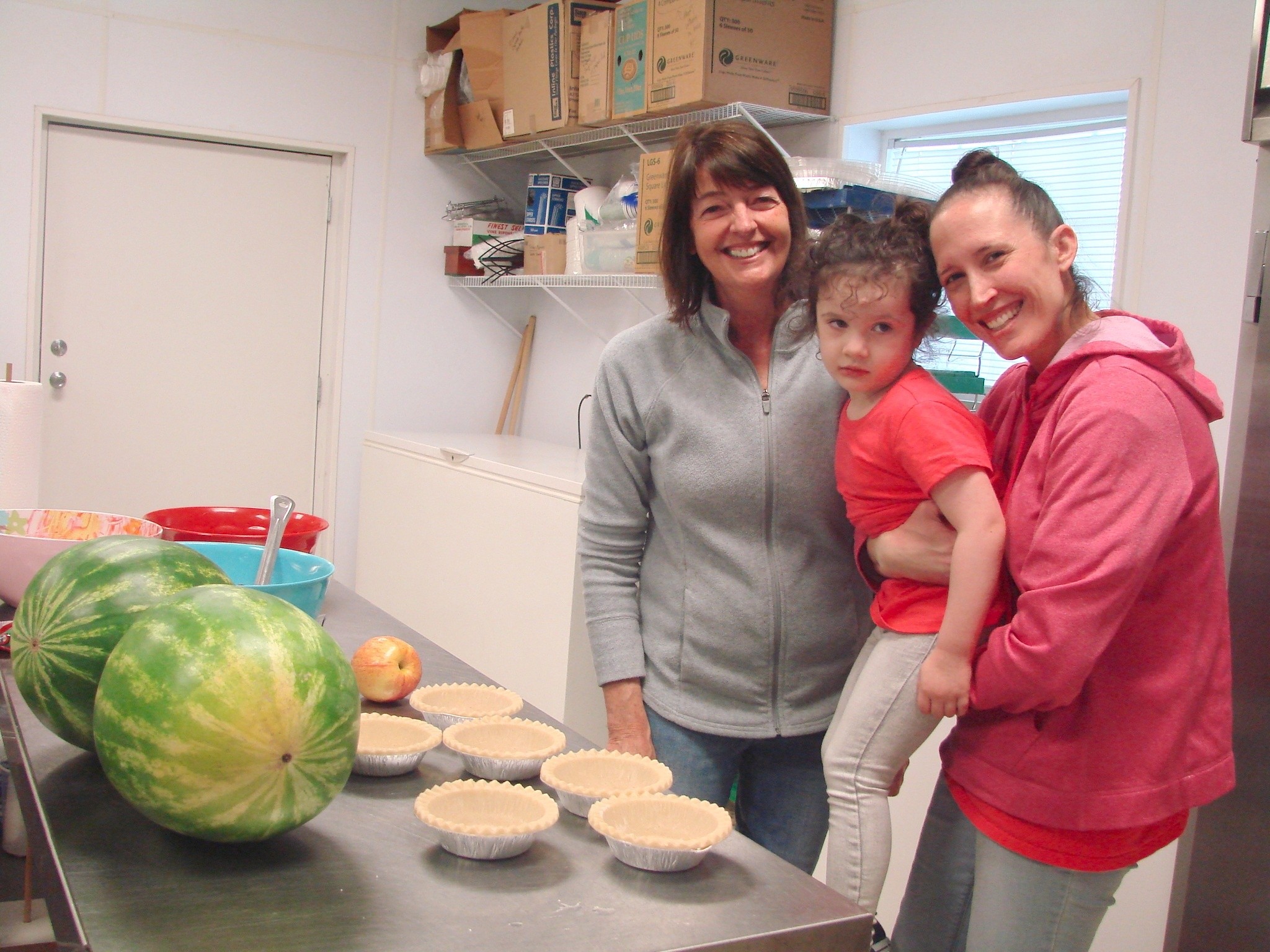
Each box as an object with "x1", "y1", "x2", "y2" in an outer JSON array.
[
  {"x1": 811, "y1": 192, "x2": 1008, "y2": 952},
  {"x1": 579, "y1": 119, "x2": 911, "y2": 882},
  {"x1": 879, "y1": 148, "x2": 1238, "y2": 952}
]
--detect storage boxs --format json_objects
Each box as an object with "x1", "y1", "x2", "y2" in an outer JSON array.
[
  {"x1": 442, "y1": 150, "x2": 676, "y2": 279},
  {"x1": 423, "y1": 0, "x2": 836, "y2": 157}
]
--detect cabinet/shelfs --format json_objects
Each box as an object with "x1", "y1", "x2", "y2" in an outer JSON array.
[{"x1": 446, "y1": 100, "x2": 834, "y2": 341}]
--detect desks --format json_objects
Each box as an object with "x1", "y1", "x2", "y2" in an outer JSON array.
[{"x1": 0, "y1": 580, "x2": 875, "y2": 952}]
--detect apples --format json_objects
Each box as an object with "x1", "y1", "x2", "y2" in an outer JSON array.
[{"x1": 351, "y1": 635, "x2": 422, "y2": 702}]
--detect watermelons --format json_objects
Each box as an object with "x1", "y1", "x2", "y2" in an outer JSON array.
[
  {"x1": 10, "y1": 534, "x2": 236, "y2": 754},
  {"x1": 93, "y1": 583, "x2": 361, "y2": 840}
]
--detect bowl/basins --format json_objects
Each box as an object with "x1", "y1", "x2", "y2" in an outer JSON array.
[
  {"x1": 169, "y1": 541, "x2": 336, "y2": 620},
  {"x1": 142, "y1": 506, "x2": 330, "y2": 555},
  {"x1": 0, "y1": 509, "x2": 163, "y2": 609}
]
